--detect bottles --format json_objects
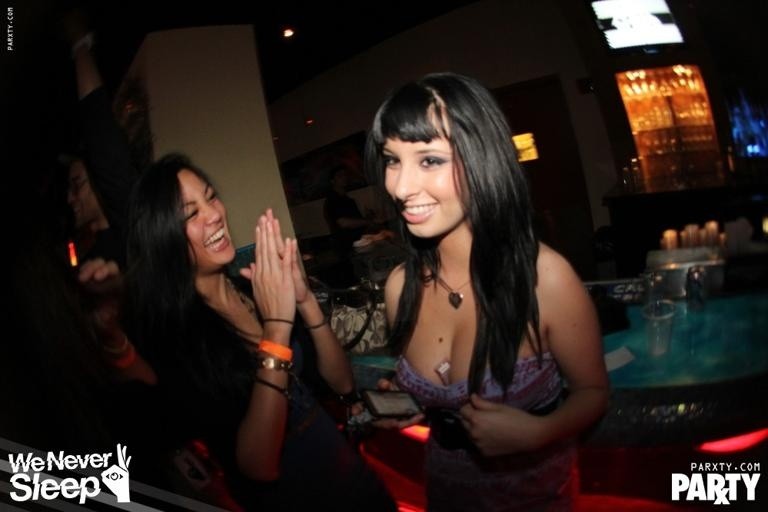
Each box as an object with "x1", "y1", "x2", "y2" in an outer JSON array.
[
  {"x1": 630, "y1": 158, "x2": 643, "y2": 189},
  {"x1": 636, "y1": 99, "x2": 709, "y2": 129},
  {"x1": 620, "y1": 165, "x2": 634, "y2": 192}
]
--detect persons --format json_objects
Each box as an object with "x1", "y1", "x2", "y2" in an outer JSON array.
[{"x1": 350, "y1": 72, "x2": 610, "y2": 512}]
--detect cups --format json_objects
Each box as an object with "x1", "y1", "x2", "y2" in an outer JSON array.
[
  {"x1": 660, "y1": 219, "x2": 726, "y2": 250},
  {"x1": 621, "y1": 78, "x2": 698, "y2": 96},
  {"x1": 643, "y1": 132, "x2": 716, "y2": 154},
  {"x1": 640, "y1": 298, "x2": 679, "y2": 356}
]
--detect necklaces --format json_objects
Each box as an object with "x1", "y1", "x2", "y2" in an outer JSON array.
[{"x1": 437, "y1": 278, "x2": 472, "y2": 310}]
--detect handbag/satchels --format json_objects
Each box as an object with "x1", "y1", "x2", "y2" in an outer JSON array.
[{"x1": 327, "y1": 288, "x2": 390, "y2": 356}]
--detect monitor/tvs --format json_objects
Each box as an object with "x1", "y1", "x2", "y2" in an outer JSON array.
[{"x1": 587, "y1": 0, "x2": 689, "y2": 51}]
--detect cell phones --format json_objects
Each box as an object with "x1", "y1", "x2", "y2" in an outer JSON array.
[{"x1": 358, "y1": 388, "x2": 425, "y2": 417}]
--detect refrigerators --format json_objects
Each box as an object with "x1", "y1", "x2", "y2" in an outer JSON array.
[{"x1": 588, "y1": 42, "x2": 735, "y2": 193}]
